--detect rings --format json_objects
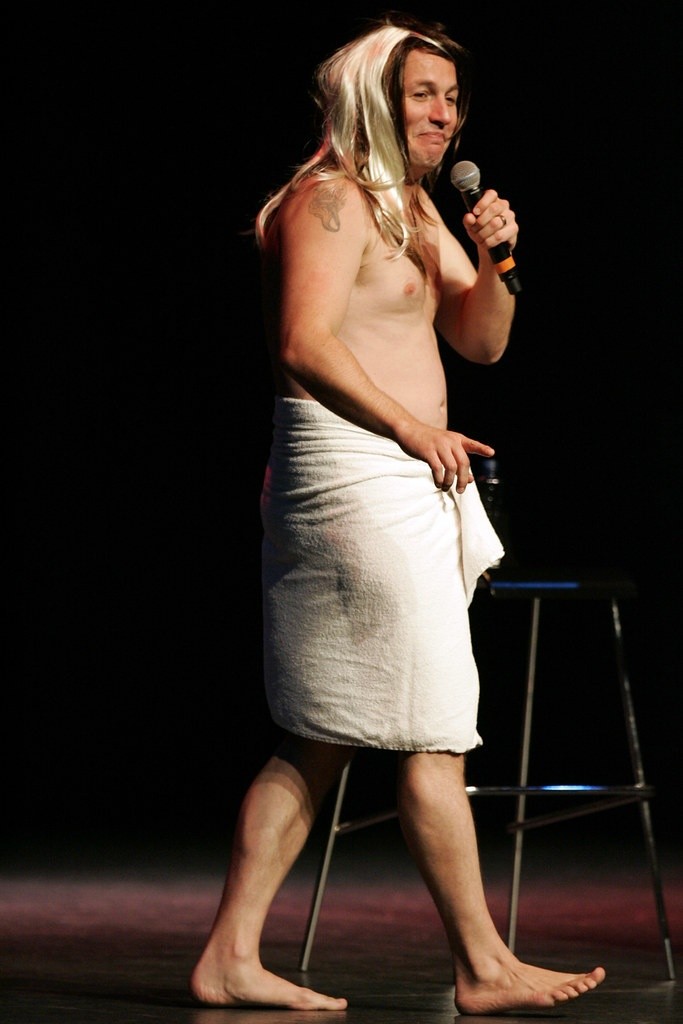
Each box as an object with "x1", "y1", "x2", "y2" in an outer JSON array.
[{"x1": 495, "y1": 215, "x2": 506, "y2": 226}]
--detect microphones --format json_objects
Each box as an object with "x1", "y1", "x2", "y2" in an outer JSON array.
[{"x1": 452, "y1": 161, "x2": 521, "y2": 295}]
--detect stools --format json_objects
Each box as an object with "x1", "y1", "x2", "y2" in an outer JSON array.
[{"x1": 301, "y1": 571, "x2": 676, "y2": 990}]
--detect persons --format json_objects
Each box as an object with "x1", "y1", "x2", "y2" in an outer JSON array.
[{"x1": 192, "y1": 26, "x2": 606, "y2": 1014}]
